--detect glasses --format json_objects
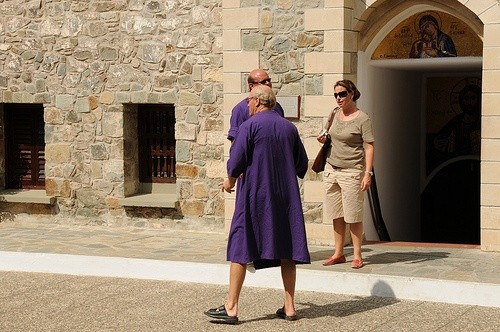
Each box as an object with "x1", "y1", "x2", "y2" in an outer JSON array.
[
  {"x1": 245, "y1": 96, "x2": 258, "y2": 104},
  {"x1": 334, "y1": 90, "x2": 352, "y2": 99},
  {"x1": 251, "y1": 78, "x2": 271, "y2": 85}
]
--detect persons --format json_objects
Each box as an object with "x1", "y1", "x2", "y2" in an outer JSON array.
[
  {"x1": 204, "y1": 85, "x2": 311, "y2": 321},
  {"x1": 317, "y1": 80, "x2": 375, "y2": 268},
  {"x1": 227, "y1": 69, "x2": 285, "y2": 265}
]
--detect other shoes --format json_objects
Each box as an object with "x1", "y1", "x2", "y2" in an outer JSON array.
[
  {"x1": 351, "y1": 258, "x2": 363, "y2": 268},
  {"x1": 322, "y1": 255, "x2": 347, "y2": 266}
]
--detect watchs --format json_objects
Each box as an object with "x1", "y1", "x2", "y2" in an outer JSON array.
[{"x1": 366, "y1": 171, "x2": 374, "y2": 176}]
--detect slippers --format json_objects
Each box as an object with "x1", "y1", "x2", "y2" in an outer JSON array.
[
  {"x1": 204, "y1": 304, "x2": 239, "y2": 324},
  {"x1": 275, "y1": 304, "x2": 298, "y2": 321}
]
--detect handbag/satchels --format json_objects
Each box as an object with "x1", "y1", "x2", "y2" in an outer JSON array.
[{"x1": 311, "y1": 107, "x2": 340, "y2": 173}]
